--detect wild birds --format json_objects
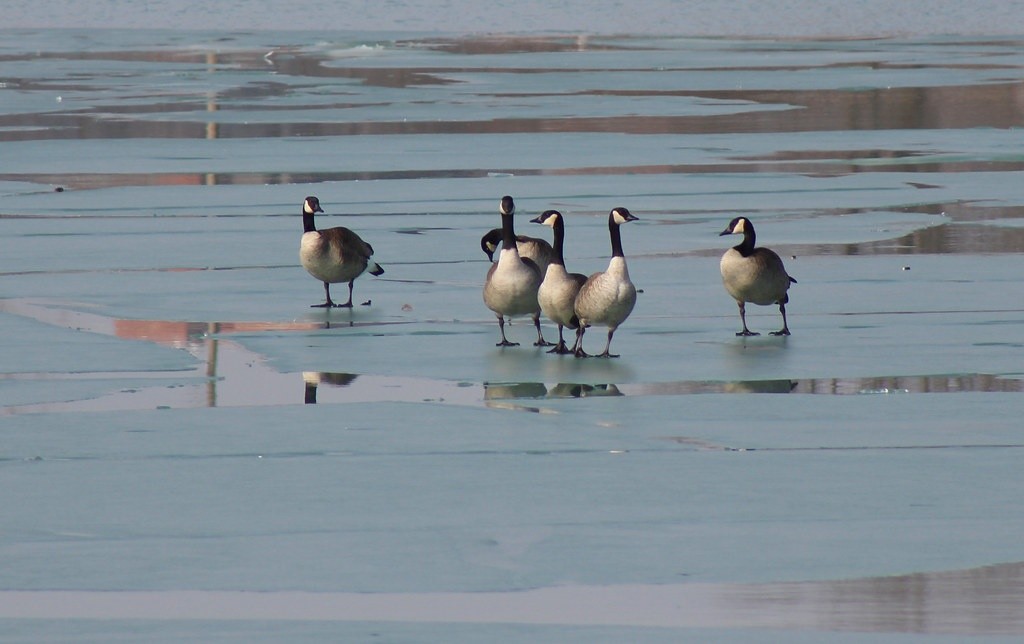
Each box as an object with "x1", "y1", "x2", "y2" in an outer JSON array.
[
  {"x1": 480, "y1": 196, "x2": 639, "y2": 360},
  {"x1": 300, "y1": 196, "x2": 385, "y2": 309},
  {"x1": 718, "y1": 216, "x2": 797, "y2": 337}
]
